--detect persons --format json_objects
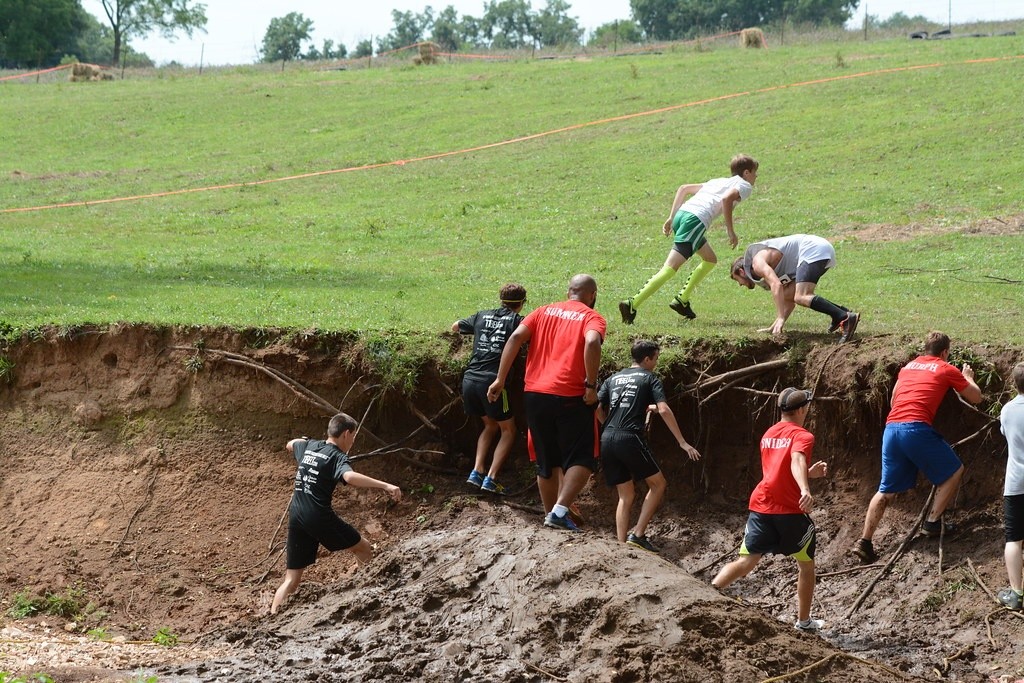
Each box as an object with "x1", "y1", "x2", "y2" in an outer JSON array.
[
  {"x1": 618, "y1": 155, "x2": 759, "y2": 325},
  {"x1": 998, "y1": 362, "x2": 1024, "y2": 610},
  {"x1": 710, "y1": 387, "x2": 828, "y2": 630},
  {"x1": 853, "y1": 332, "x2": 982, "y2": 563},
  {"x1": 271, "y1": 414, "x2": 401, "y2": 614},
  {"x1": 598, "y1": 341, "x2": 701, "y2": 554},
  {"x1": 731, "y1": 233, "x2": 860, "y2": 344},
  {"x1": 487, "y1": 274, "x2": 606, "y2": 531},
  {"x1": 452, "y1": 284, "x2": 527, "y2": 494}
]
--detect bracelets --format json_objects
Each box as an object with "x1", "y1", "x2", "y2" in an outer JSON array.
[{"x1": 584, "y1": 378, "x2": 599, "y2": 388}]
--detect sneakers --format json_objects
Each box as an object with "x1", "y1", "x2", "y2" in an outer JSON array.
[
  {"x1": 481, "y1": 476, "x2": 508, "y2": 495},
  {"x1": 827, "y1": 306, "x2": 851, "y2": 333},
  {"x1": 626, "y1": 533, "x2": 660, "y2": 554},
  {"x1": 467, "y1": 470, "x2": 484, "y2": 486},
  {"x1": 839, "y1": 311, "x2": 860, "y2": 344},
  {"x1": 619, "y1": 299, "x2": 637, "y2": 326},
  {"x1": 544, "y1": 509, "x2": 582, "y2": 532},
  {"x1": 670, "y1": 296, "x2": 697, "y2": 319},
  {"x1": 997, "y1": 588, "x2": 1024, "y2": 611},
  {"x1": 793, "y1": 619, "x2": 826, "y2": 630},
  {"x1": 922, "y1": 519, "x2": 949, "y2": 536},
  {"x1": 852, "y1": 537, "x2": 875, "y2": 562}
]
